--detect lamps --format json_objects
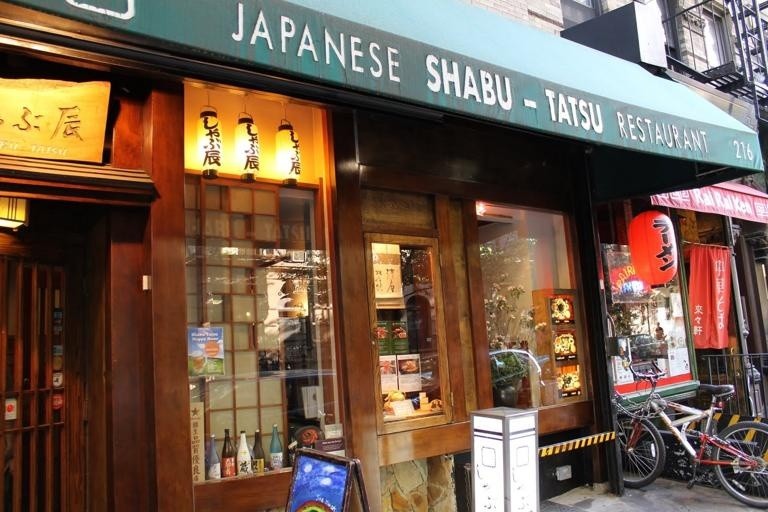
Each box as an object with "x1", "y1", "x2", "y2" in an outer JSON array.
[
  {"x1": 276, "y1": 101, "x2": 301, "y2": 186},
  {"x1": 235, "y1": 94, "x2": 259, "y2": 182},
  {"x1": 198, "y1": 85, "x2": 223, "y2": 179}
]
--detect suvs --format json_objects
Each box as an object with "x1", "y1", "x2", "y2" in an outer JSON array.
[{"x1": 415, "y1": 344, "x2": 548, "y2": 411}]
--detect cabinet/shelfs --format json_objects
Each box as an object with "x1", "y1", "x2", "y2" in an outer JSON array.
[{"x1": 531, "y1": 287, "x2": 588, "y2": 406}]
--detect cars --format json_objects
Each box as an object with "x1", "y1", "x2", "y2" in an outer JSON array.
[
  {"x1": 257, "y1": 315, "x2": 309, "y2": 373},
  {"x1": 615, "y1": 334, "x2": 670, "y2": 361},
  {"x1": 188, "y1": 364, "x2": 333, "y2": 446}
]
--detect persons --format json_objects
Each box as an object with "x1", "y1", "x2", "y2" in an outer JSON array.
[{"x1": 655, "y1": 322, "x2": 663, "y2": 332}]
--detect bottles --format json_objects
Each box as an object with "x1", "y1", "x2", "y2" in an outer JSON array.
[
  {"x1": 222, "y1": 428, "x2": 236, "y2": 478},
  {"x1": 287, "y1": 425, "x2": 303, "y2": 466},
  {"x1": 206, "y1": 434, "x2": 221, "y2": 479},
  {"x1": 270, "y1": 423, "x2": 285, "y2": 468},
  {"x1": 252, "y1": 429, "x2": 264, "y2": 473},
  {"x1": 236, "y1": 429, "x2": 251, "y2": 474}
]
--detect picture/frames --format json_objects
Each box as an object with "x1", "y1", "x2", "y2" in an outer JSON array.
[{"x1": 285, "y1": 447, "x2": 371, "y2": 512}]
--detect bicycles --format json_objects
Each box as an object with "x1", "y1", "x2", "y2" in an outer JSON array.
[{"x1": 614, "y1": 357, "x2": 768, "y2": 511}]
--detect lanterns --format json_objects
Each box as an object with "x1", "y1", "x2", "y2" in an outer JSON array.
[
  {"x1": 236, "y1": 111, "x2": 260, "y2": 185},
  {"x1": 627, "y1": 210, "x2": 679, "y2": 289},
  {"x1": 274, "y1": 118, "x2": 301, "y2": 185},
  {"x1": 197, "y1": 105, "x2": 222, "y2": 179}
]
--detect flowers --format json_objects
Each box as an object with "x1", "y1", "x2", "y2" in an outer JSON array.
[{"x1": 483, "y1": 282, "x2": 546, "y2": 388}]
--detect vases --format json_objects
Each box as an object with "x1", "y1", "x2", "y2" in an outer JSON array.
[{"x1": 493, "y1": 381, "x2": 517, "y2": 406}]
